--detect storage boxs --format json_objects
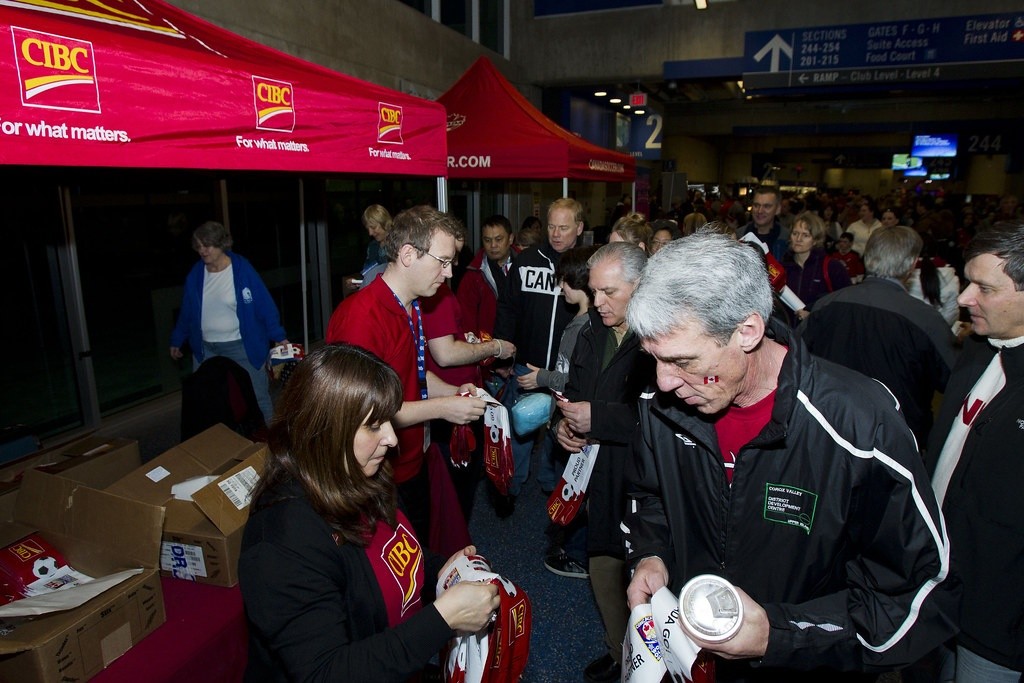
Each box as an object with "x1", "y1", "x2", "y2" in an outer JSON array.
[
  {"x1": 102, "y1": 421, "x2": 275, "y2": 587},
  {"x1": 0, "y1": 466, "x2": 168, "y2": 683},
  {"x1": 0, "y1": 435, "x2": 142, "y2": 544}
]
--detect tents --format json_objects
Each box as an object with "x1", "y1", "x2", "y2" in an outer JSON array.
[
  {"x1": 0, "y1": 0, "x2": 447, "y2": 215},
  {"x1": 296, "y1": 56, "x2": 636, "y2": 355}
]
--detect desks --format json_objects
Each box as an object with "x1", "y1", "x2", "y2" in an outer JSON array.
[{"x1": 85, "y1": 574, "x2": 251, "y2": 683}]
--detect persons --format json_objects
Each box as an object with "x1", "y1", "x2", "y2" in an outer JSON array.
[{"x1": 172, "y1": 182, "x2": 1024, "y2": 683}]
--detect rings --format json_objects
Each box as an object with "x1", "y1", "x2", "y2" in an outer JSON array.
[{"x1": 488, "y1": 611, "x2": 497, "y2": 622}]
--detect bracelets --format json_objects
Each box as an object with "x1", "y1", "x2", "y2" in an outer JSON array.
[{"x1": 493, "y1": 338, "x2": 502, "y2": 358}]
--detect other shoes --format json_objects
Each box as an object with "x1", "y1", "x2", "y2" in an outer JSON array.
[
  {"x1": 544, "y1": 555, "x2": 589, "y2": 578},
  {"x1": 496, "y1": 493, "x2": 519, "y2": 516},
  {"x1": 583, "y1": 653, "x2": 622, "y2": 683}
]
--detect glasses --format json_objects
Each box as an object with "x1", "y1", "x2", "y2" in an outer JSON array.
[{"x1": 411, "y1": 243, "x2": 455, "y2": 267}]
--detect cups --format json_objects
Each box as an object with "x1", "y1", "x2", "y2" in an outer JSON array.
[{"x1": 678, "y1": 574, "x2": 744, "y2": 644}]
